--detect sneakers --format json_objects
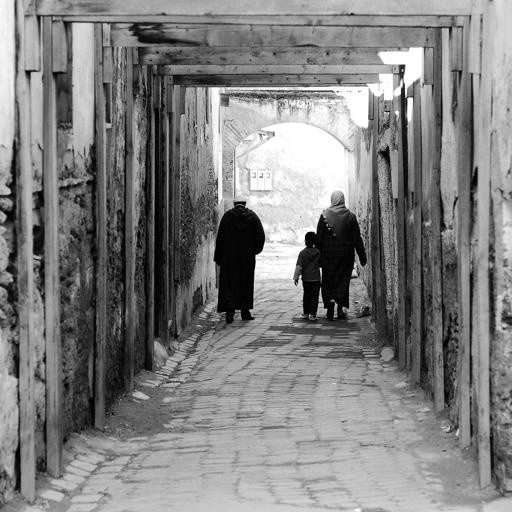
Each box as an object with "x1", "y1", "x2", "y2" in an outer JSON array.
[
  {"x1": 302, "y1": 313, "x2": 309, "y2": 319},
  {"x1": 327, "y1": 302, "x2": 334, "y2": 319},
  {"x1": 309, "y1": 313, "x2": 318, "y2": 320},
  {"x1": 338, "y1": 311, "x2": 345, "y2": 316}
]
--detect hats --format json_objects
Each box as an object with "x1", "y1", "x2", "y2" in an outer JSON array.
[{"x1": 233, "y1": 196, "x2": 247, "y2": 203}]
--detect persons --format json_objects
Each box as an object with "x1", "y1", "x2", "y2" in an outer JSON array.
[
  {"x1": 315, "y1": 190, "x2": 367, "y2": 320},
  {"x1": 213, "y1": 196, "x2": 265, "y2": 324},
  {"x1": 293, "y1": 231, "x2": 321, "y2": 321}
]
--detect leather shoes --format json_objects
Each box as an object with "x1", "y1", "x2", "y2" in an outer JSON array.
[{"x1": 243, "y1": 315, "x2": 254, "y2": 320}]
